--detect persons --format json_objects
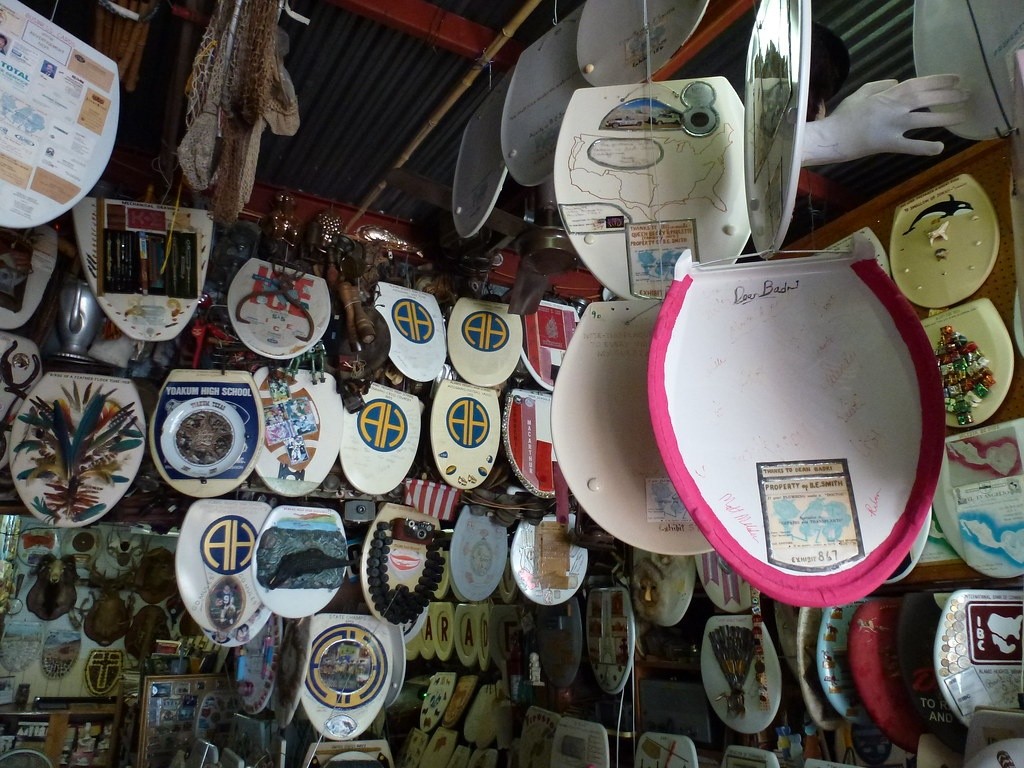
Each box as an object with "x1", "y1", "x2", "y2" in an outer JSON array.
[
  {"x1": 41, "y1": 64, "x2": 54, "y2": 79},
  {"x1": 799, "y1": 74, "x2": 972, "y2": 165},
  {"x1": 0, "y1": 34, "x2": 7, "y2": 55}
]
